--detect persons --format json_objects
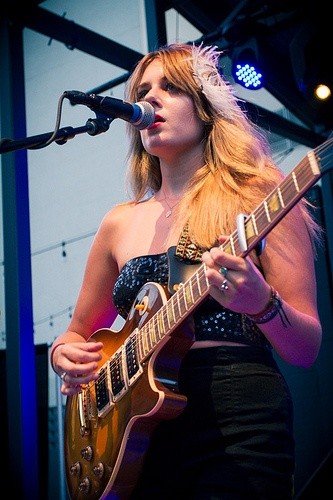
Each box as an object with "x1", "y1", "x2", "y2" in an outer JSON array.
[{"x1": 48, "y1": 43, "x2": 322, "y2": 500}]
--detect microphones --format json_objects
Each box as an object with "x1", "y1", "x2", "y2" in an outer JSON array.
[{"x1": 62, "y1": 90, "x2": 156, "y2": 130}]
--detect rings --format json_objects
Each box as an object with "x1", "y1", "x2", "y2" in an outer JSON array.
[
  {"x1": 59, "y1": 372, "x2": 68, "y2": 381},
  {"x1": 218, "y1": 280, "x2": 229, "y2": 292},
  {"x1": 218, "y1": 266, "x2": 229, "y2": 277}
]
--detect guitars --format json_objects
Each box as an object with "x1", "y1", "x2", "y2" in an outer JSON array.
[{"x1": 66, "y1": 137, "x2": 333, "y2": 500}]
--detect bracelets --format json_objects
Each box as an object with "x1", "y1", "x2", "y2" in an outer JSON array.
[
  {"x1": 245, "y1": 284, "x2": 293, "y2": 329},
  {"x1": 50, "y1": 342, "x2": 66, "y2": 378}
]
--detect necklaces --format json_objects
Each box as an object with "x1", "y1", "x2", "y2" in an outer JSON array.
[{"x1": 157, "y1": 185, "x2": 192, "y2": 218}]
